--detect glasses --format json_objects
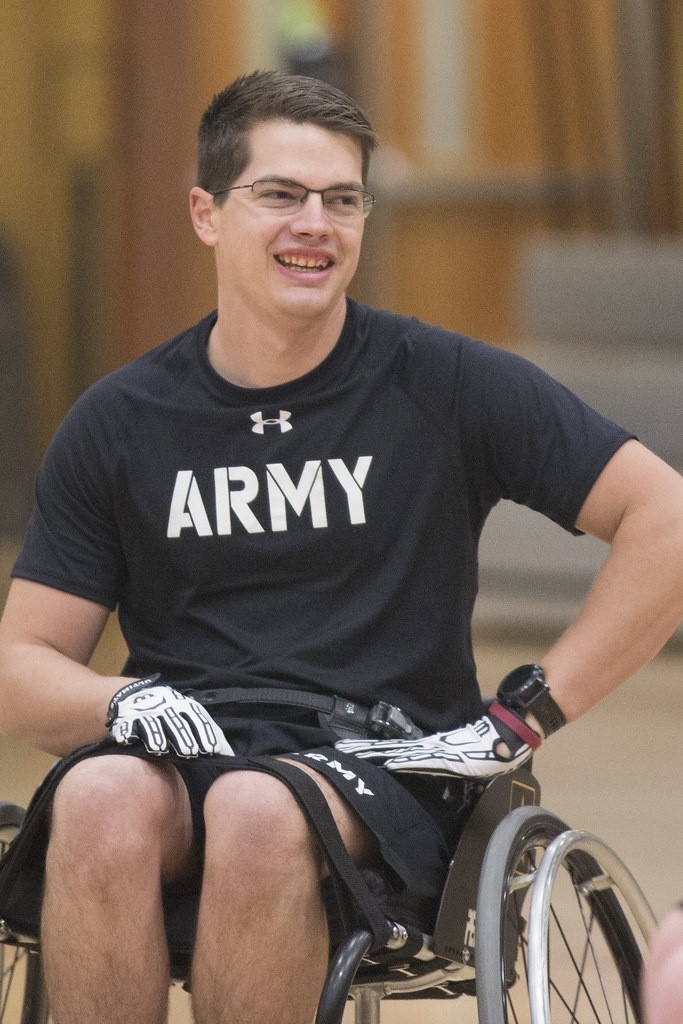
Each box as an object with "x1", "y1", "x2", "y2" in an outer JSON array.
[{"x1": 210, "y1": 179, "x2": 377, "y2": 221}]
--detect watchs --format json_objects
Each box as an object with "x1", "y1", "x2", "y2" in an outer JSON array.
[{"x1": 496, "y1": 663, "x2": 568, "y2": 739}]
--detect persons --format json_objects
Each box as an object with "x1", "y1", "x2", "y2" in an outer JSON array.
[{"x1": 0, "y1": 68, "x2": 683, "y2": 1024}]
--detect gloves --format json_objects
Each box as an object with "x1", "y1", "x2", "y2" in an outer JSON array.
[
  {"x1": 103, "y1": 673, "x2": 237, "y2": 760},
  {"x1": 333, "y1": 699, "x2": 543, "y2": 779}
]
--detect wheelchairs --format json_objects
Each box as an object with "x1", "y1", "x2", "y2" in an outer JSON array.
[{"x1": 0, "y1": 699, "x2": 661, "y2": 1024}]
